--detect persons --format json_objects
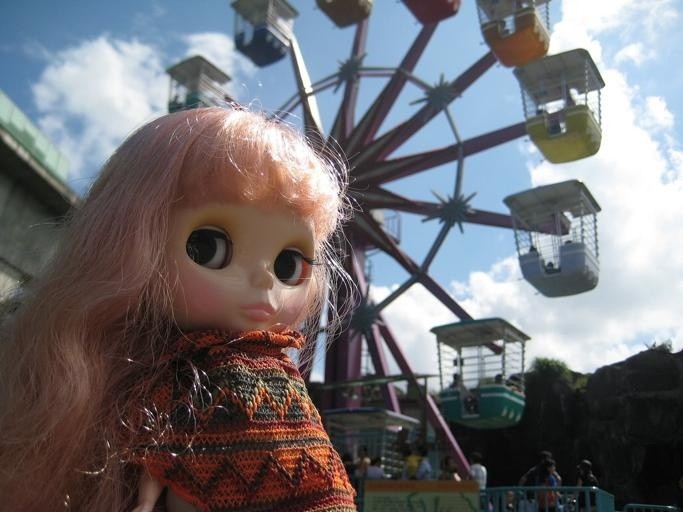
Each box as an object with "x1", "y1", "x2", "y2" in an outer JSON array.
[
  {"x1": 342, "y1": 443, "x2": 487, "y2": 511},
  {"x1": 2, "y1": 105, "x2": 362, "y2": 512},
  {"x1": 536, "y1": 96, "x2": 576, "y2": 136},
  {"x1": 488, "y1": 451, "x2": 600, "y2": 510},
  {"x1": 450, "y1": 373, "x2": 523, "y2": 413},
  {"x1": 529, "y1": 240, "x2": 573, "y2": 274}
]
